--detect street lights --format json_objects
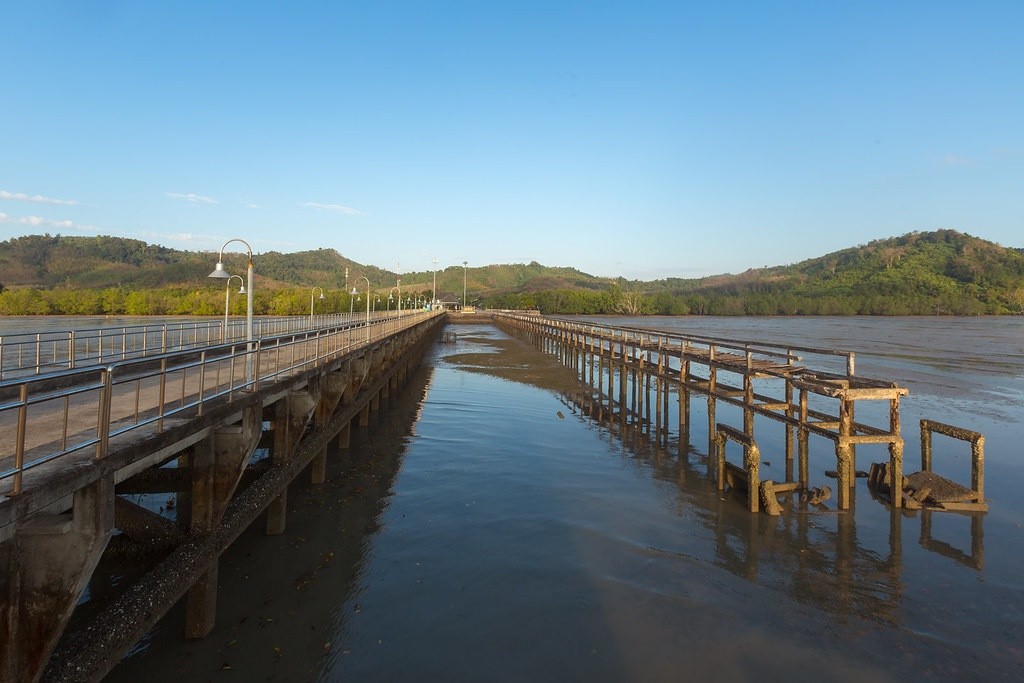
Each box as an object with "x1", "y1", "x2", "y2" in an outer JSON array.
[
  {"x1": 207, "y1": 239, "x2": 252, "y2": 390},
  {"x1": 351, "y1": 293, "x2": 361, "y2": 320},
  {"x1": 311, "y1": 286, "x2": 324, "y2": 330},
  {"x1": 387, "y1": 287, "x2": 401, "y2": 319},
  {"x1": 350, "y1": 276, "x2": 371, "y2": 343},
  {"x1": 387, "y1": 295, "x2": 431, "y2": 316},
  {"x1": 372, "y1": 294, "x2": 381, "y2": 315},
  {"x1": 224, "y1": 274, "x2": 246, "y2": 343}
]
